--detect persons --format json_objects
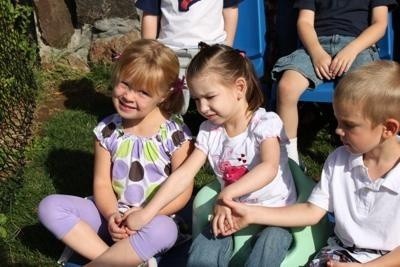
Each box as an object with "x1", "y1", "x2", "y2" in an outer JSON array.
[
  {"x1": 134, "y1": 0, "x2": 241, "y2": 136},
  {"x1": 38, "y1": 40, "x2": 194, "y2": 267},
  {"x1": 120, "y1": 41, "x2": 297, "y2": 267},
  {"x1": 272, "y1": 0, "x2": 388, "y2": 165},
  {"x1": 207, "y1": 61, "x2": 400, "y2": 267}
]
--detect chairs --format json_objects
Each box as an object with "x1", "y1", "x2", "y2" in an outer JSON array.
[
  {"x1": 265, "y1": 9, "x2": 393, "y2": 113},
  {"x1": 231, "y1": 1, "x2": 263, "y2": 81}
]
